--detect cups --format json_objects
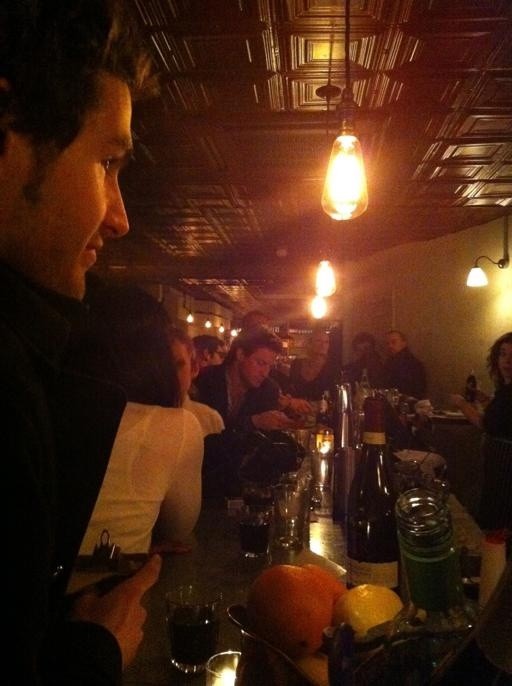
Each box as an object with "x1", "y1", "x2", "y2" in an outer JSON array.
[
  {"x1": 206, "y1": 651, "x2": 252, "y2": 686},
  {"x1": 310, "y1": 433, "x2": 333, "y2": 516},
  {"x1": 390, "y1": 486, "x2": 459, "y2": 609},
  {"x1": 240, "y1": 481, "x2": 274, "y2": 556},
  {"x1": 165, "y1": 583, "x2": 224, "y2": 675},
  {"x1": 391, "y1": 461, "x2": 449, "y2": 503},
  {"x1": 294, "y1": 429, "x2": 308, "y2": 449},
  {"x1": 269, "y1": 470, "x2": 312, "y2": 552}
]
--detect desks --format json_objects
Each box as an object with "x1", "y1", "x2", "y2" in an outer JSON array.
[
  {"x1": 117, "y1": 400, "x2": 507, "y2": 686},
  {"x1": 407, "y1": 410, "x2": 483, "y2": 425}
]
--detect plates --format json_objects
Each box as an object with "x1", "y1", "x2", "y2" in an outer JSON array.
[
  {"x1": 394, "y1": 449, "x2": 447, "y2": 474},
  {"x1": 441, "y1": 410, "x2": 463, "y2": 417}
]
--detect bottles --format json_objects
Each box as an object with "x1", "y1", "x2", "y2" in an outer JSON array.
[
  {"x1": 319, "y1": 394, "x2": 328, "y2": 420},
  {"x1": 333, "y1": 379, "x2": 403, "y2": 589},
  {"x1": 478, "y1": 539, "x2": 507, "y2": 611},
  {"x1": 465, "y1": 371, "x2": 481, "y2": 402}
]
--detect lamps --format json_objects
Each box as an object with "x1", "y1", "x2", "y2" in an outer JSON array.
[
  {"x1": 466, "y1": 255, "x2": 506, "y2": 287},
  {"x1": 186, "y1": 295, "x2": 237, "y2": 336},
  {"x1": 204, "y1": 650, "x2": 241, "y2": 686},
  {"x1": 316, "y1": 426, "x2": 335, "y2": 455},
  {"x1": 319, "y1": 1, "x2": 368, "y2": 221}
]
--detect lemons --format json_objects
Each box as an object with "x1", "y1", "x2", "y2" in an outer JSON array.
[{"x1": 333, "y1": 582, "x2": 404, "y2": 641}]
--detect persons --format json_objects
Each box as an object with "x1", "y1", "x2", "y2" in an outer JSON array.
[
  {"x1": 0, "y1": 0, "x2": 163, "y2": 686},
  {"x1": 78, "y1": 285, "x2": 341, "y2": 564},
  {"x1": 342, "y1": 329, "x2": 425, "y2": 413},
  {"x1": 439, "y1": 333, "x2": 512, "y2": 438}
]
